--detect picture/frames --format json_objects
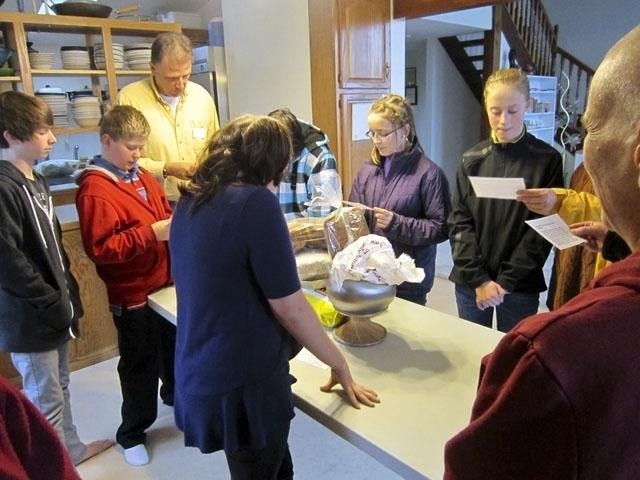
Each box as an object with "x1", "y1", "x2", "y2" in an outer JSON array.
[{"x1": 405, "y1": 67, "x2": 417, "y2": 104}]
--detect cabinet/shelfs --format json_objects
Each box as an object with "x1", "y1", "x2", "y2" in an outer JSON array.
[
  {"x1": 0, "y1": 11, "x2": 183, "y2": 392},
  {"x1": 523, "y1": 74, "x2": 557, "y2": 148}
]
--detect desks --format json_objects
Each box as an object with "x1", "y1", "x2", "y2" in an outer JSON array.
[{"x1": 147, "y1": 270, "x2": 508, "y2": 480}]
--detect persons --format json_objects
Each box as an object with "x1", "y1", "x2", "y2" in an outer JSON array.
[
  {"x1": 348, "y1": 95, "x2": 452, "y2": 307},
  {"x1": 515, "y1": 162, "x2": 614, "y2": 312},
  {"x1": 172, "y1": 116, "x2": 380, "y2": 480},
  {"x1": 268, "y1": 109, "x2": 342, "y2": 224},
  {"x1": 0, "y1": 91, "x2": 116, "y2": 467},
  {"x1": 71, "y1": 105, "x2": 173, "y2": 467},
  {"x1": 567, "y1": 221, "x2": 632, "y2": 262},
  {"x1": 446, "y1": 68, "x2": 564, "y2": 335},
  {"x1": 443, "y1": 23, "x2": 640, "y2": 480},
  {"x1": 115, "y1": 31, "x2": 220, "y2": 212}
]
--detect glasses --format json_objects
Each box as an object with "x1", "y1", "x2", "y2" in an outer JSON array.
[{"x1": 364, "y1": 124, "x2": 406, "y2": 140}]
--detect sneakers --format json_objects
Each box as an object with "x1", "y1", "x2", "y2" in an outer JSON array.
[{"x1": 115, "y1": 441, "x2": 150, "y2": 467}]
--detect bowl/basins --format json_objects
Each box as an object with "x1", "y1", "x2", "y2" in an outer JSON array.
[
  {"x1": 0, "y1": 46, "x2": 14, "y2": 67},
  {"x1": 28, "y1": 52, "x2": 55, "y2": 70}
]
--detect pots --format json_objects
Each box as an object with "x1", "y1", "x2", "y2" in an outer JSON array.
[
  {"x1": 49, "y1": 0, "x2": 141, "y2": 18},
  {"x1": 66, "y1": 84, "x2": 109, "y2": 100}
]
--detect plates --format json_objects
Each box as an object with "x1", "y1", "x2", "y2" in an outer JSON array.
[
  {"x1": 35, "y1": 87, "x2": 69, "y2": 128},
  {"x1": 71, "y1": 95, "x2": 103, "y2": 128},
  {"x1": 60, "y1": 45, "x2": 91, "y2": 70},
  {"x1": 93, "y1": 43, "x2": 125, "y2": 70},
  {"x1": 124, "y1": 42, "x2": 154, "y2": 71}
]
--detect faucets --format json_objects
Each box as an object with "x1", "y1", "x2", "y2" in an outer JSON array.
[{"x1": 74, "y1": 144, "x2": 79, "y2": 160}]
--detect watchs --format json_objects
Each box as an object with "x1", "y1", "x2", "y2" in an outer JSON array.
[{"x1": 162, "y1": 169, "x2": 168, "y2": 179}]
